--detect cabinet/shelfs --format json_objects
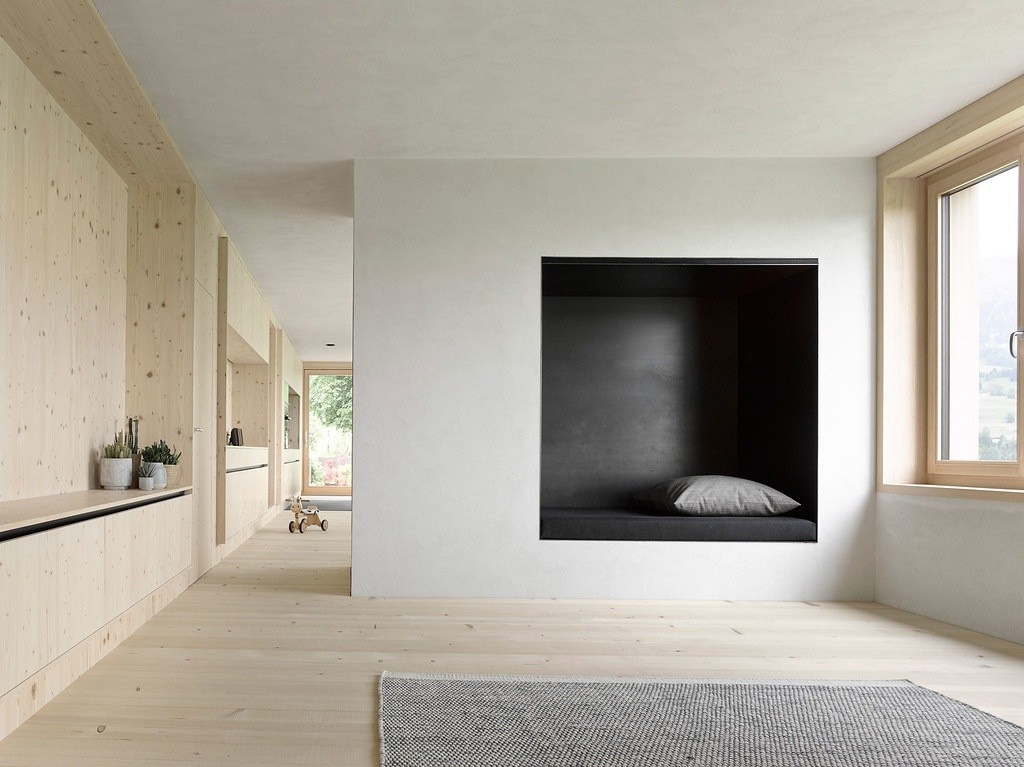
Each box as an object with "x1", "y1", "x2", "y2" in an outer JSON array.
[{"x1": 0, "y1": 0, "x2": 303, "y2": 740}]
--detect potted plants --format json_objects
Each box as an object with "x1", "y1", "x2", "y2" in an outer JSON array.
[
  {"x1": 100, "y1": 430, "x2": 132, "y2": 489},
  {"x1": 136, "y1": 464, "x2": 156, "y2": 490},
  {"x1": 141, "y1": 441, "x2": 168, "y2": 490},
  {"x1": 159, "y1": 440, "x2": 182, "y2": 487},
  {"x1": 129, "y1": 416, "x2": 141, "y2": 486}
]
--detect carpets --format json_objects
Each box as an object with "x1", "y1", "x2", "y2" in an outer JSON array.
[{"x1": 379, "y1": 668, "x2": 1024, "y2": 767}]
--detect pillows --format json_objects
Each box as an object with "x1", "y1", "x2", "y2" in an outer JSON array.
[{"x1": 633, "y1": 475, "x2": 801, "y2": 516}]
